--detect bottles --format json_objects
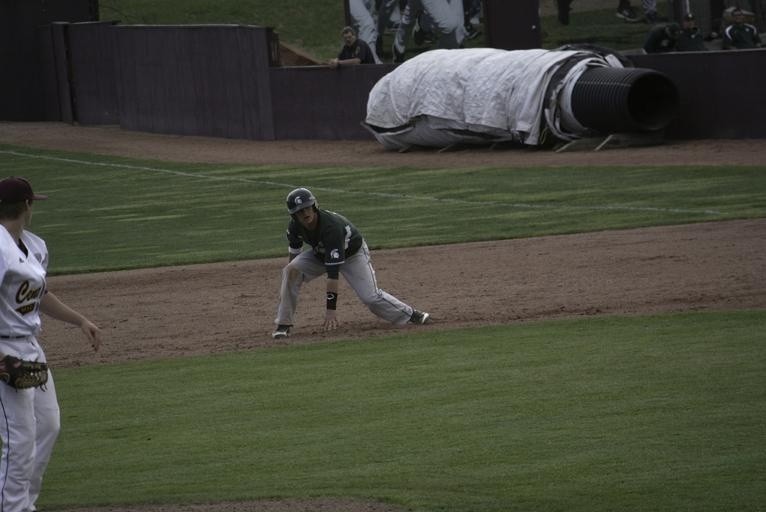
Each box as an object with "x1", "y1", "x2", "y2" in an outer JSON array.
[{"x1": 0, "y1": 175, "x2": 48, "y2": 201}]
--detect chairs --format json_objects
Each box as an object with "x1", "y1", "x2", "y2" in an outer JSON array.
[
  {"x1": 411, "y1": 310, "x2": 429, "y2": 324},
  {"x1": 271, "y1": 325, "x2": 289, "y2": 339}
]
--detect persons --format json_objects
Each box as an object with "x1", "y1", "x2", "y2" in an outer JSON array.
[
  {"x1": 325, "y1": 27, "x2": 376, "y2": 66},
  {"x1": 0, "y1": 177, "x2": 105, "y2": 512},
  {"x1": 270, "y1": 186, "x2": 430, "y2": 338},
  {"x1": 350, "y1": 0, "x2": 485, "y2": 65},
  {"x1": 614, "y1": 0, "x2": 764, "y2": 55}
]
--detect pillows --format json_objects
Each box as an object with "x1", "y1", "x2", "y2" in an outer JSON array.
[{"x1": 286, "y1": 187, "x2": 315, "y2": 214}]
